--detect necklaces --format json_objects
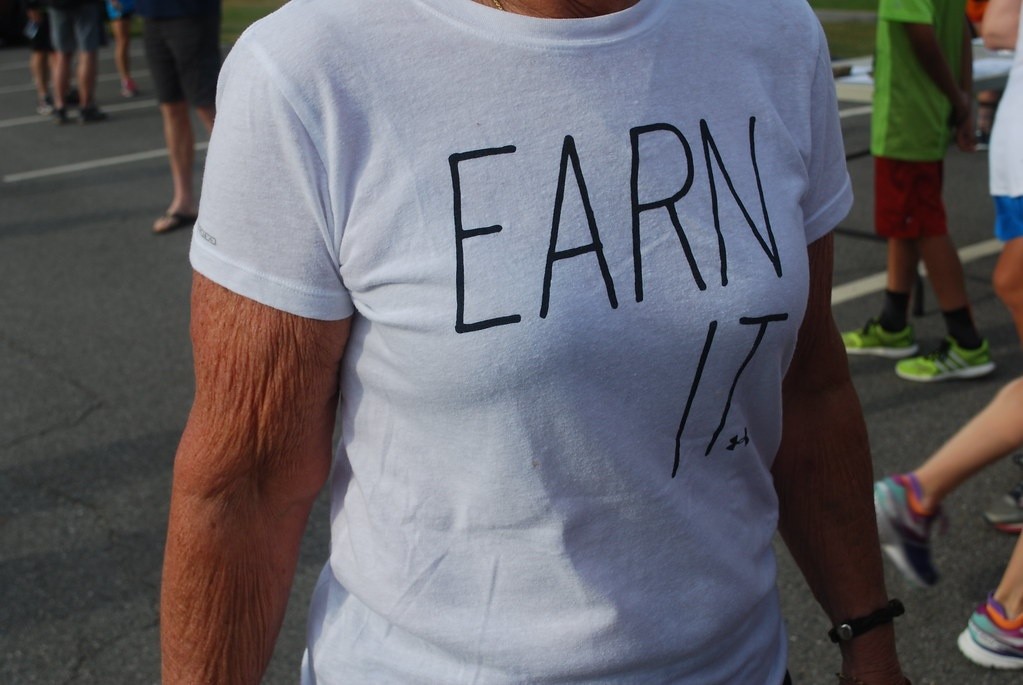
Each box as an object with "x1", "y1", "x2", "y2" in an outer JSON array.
[{"x1": 493, "y1": 0, "x2": 503, "y2": 10}]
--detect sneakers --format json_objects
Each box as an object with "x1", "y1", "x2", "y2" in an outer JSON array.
[
  {"x1": 840, "y1": 314, "x2": 919, "y2": 358},
  {"x1": 984, "y1": 487, "x2": 1023, "y2": 533},
  {"x1": 956, "y1": 604, "x2": 1023, "y2": 668},
  {"x1": 873, "y1": 477, "x2": 939, "y2": 587},
  {"x1": 895, "y1": 335, "x2": 996, "y2": 381}
]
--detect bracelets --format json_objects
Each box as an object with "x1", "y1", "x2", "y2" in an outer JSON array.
[
  {"x1": 828, "y1": 597, "x2": 906, "y2": 643},
  {"x1": 160, "y1": 0, "x2": 915, "y2": 685}
]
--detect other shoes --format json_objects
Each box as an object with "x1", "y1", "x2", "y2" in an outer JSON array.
[
  {"x1": 80, "y1": 106, "x2": 107, "y2": 120},
  {"x1": 53, "y1": 108, "x2": 67, "y2": 120}
]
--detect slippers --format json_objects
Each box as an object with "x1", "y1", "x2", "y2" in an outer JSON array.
[{"x1": 154, "y1": 210, "x2": 197, "y2": 233}]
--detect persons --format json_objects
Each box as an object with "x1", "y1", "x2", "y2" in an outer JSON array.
[
  {"x1": 840, "y1": 0, "x2": 1023, "y2": 685},
  {"x1": 142, "y1": 0, "x2": 223, "y2": 237},
  {"x1": 24, "y1": 0, "x2": 145, "y2": 127}
]
